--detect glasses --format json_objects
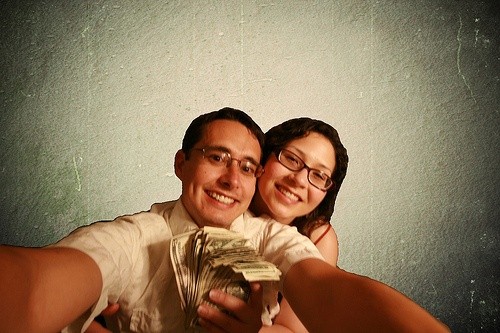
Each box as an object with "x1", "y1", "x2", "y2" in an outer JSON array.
[
  {"x1": 185, "y1": 146, "x2": 265, "y2": 177},
  {"x1": 270, "y1": 145, "x2": 333, "y2": 192}
]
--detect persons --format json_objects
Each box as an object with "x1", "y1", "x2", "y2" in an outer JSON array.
[
  {"x1": 0, "y1": 107, "x2": 452, "y2": 332},
  {"x1": 82, "y1": 117, "x2": 348, "y2": 333}
]
